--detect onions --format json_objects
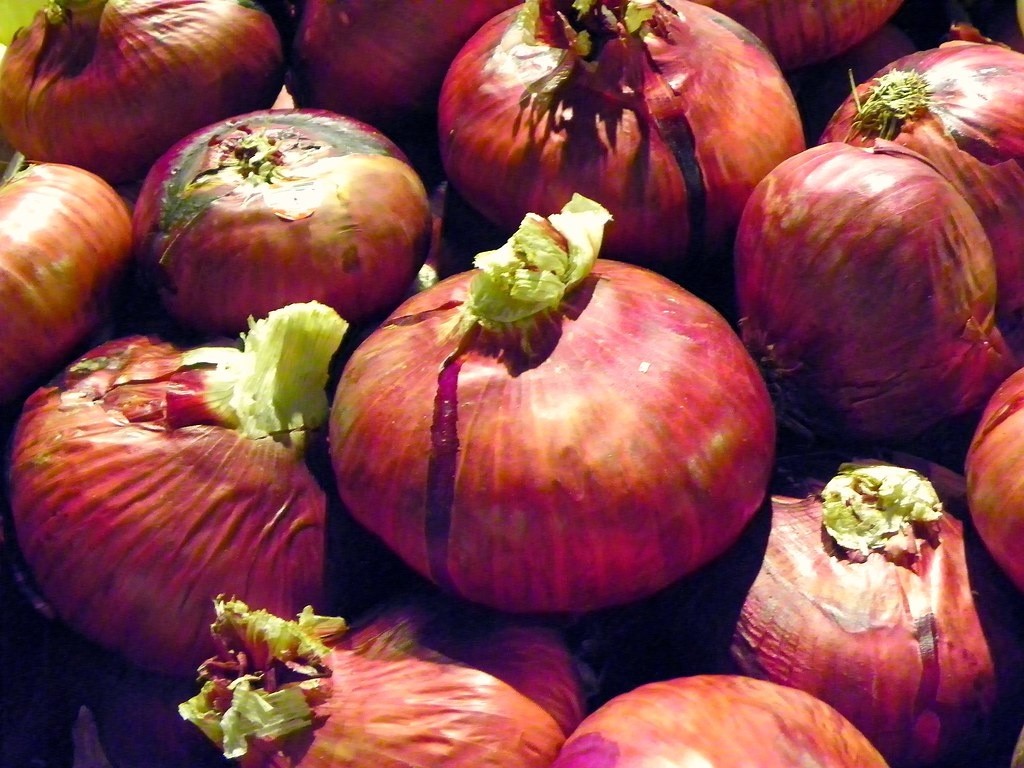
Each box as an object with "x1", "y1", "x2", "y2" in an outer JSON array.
[{"x1": 0, "y1": 0, "x2": 1023, "y2": 768}]
